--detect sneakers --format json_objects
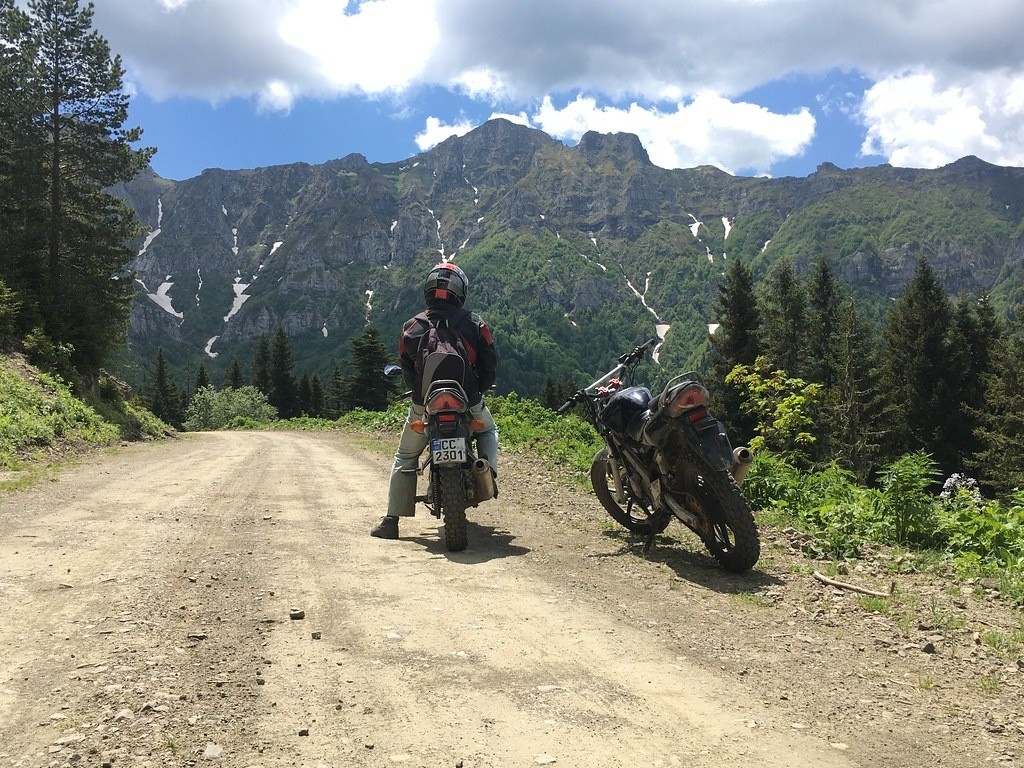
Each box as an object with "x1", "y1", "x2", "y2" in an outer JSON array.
[
  {"x1": 479, "y1": 457, "x2": 497, "y2": 478},
  {"x1": 371, "y1": 517, "x2": 399, "y2": 538}
]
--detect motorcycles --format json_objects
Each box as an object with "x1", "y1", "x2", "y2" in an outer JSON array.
[
  {"x1": 383, "y1": 361, "x2": 498, "y2": 552},
  {"x1": 557, "y1": 337, "x2": 761, "y2": 574}
]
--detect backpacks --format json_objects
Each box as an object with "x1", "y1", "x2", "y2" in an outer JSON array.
[{"x1": 414, "y1": 310, "x2": 471, "y2": 402}]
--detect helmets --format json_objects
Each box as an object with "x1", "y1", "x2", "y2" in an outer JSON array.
[{"x1": 424, "y1": 263, "x2": 468, "y2": 308}]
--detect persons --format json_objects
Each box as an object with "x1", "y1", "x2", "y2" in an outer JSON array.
[{"x1": 369, "y1": 261, "x2": 500, "y2": 540}]
